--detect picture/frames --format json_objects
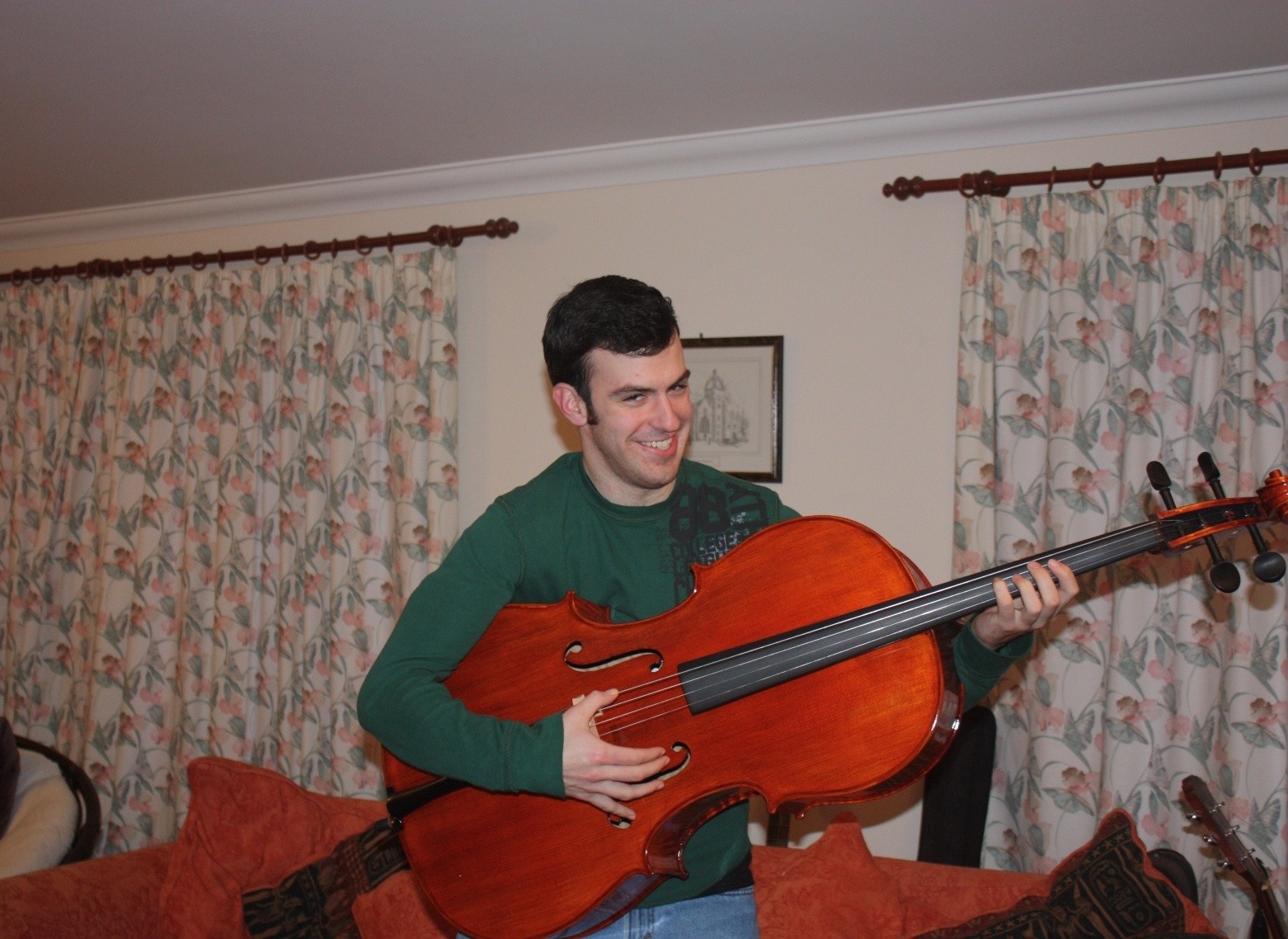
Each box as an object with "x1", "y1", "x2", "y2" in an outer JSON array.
[{"x1": 679, "y1": 335, "x2": 784, "y2": 483}]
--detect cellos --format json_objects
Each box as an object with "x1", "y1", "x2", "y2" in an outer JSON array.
[{"x1": 382, "y1": 450, "x2": 1288, "y2": 939}]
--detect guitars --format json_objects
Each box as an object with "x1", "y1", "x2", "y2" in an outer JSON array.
[{"x1": 1180, "y1": 773, "x2": 1288, "y2": 939}]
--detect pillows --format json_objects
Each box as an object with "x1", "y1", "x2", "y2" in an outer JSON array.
[
  {"x1": 751, "y1": 809, "x2": 903, "y2": 939},
  {"x1": 142, "y1": 755, "x2": 456, "y2": 939},
  {"x1": 910, "y1": 806, "x2": 1231, "y2": 939}
]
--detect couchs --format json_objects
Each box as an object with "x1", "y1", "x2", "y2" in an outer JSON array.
[{"x1": 0, "y1": 756, "x2": 1232, "y2": 939}]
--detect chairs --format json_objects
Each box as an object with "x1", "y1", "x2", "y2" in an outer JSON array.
[{"x1": 0, "y1": 714, "x2": 103, "y2": 881}]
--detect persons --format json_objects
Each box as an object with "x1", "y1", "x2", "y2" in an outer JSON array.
[{"x1": 355, "y1": 275, "x2": 1078, "y2": 939}]
